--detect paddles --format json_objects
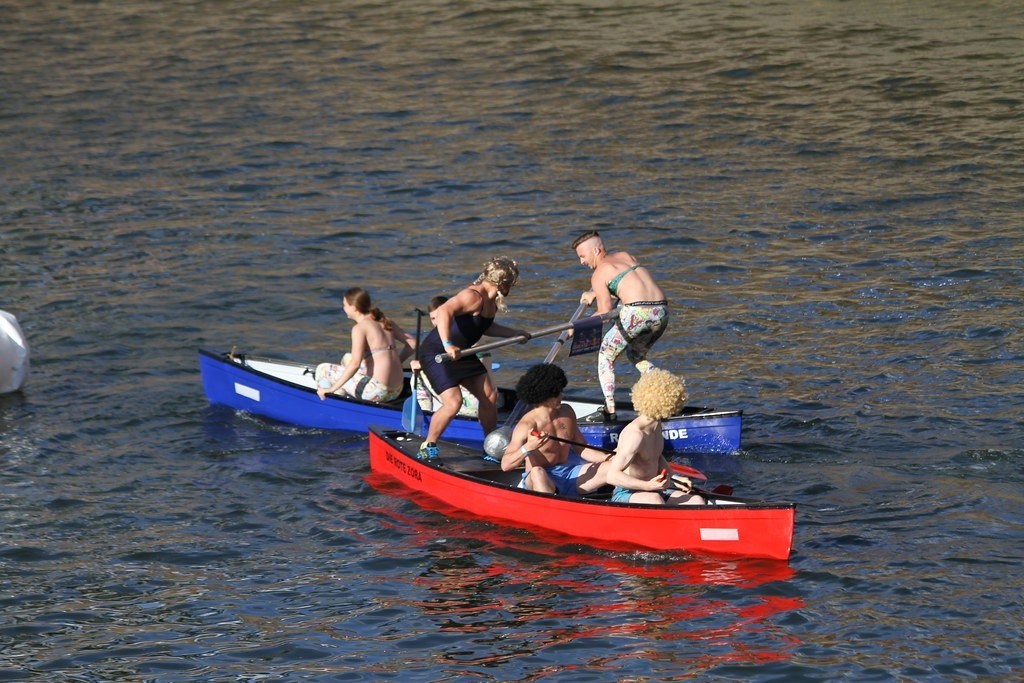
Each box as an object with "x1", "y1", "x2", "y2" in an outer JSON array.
[{"x1": 529, "y1": 429, "x2": 708, "y2": 480}]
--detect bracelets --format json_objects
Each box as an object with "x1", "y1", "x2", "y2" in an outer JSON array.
[
  {"x1": 671, "y1": 474, "x2": 678, "y2": 479},
  {"x1": 520, "y1": 445, "x2": 529, "y2": 456},
  {"x1": 443, "y1": 341, "x2": 452, "y2": 347}
]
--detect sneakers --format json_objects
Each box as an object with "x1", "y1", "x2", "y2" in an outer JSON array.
[
  {"x1": 481, "y1": 450, "x2": 501, "y2": 464},
  {"x1": 586, "y1": 407, "x2": 617, "y2": 423},
  {"x1": 416, "y1": 442, "x2": 440, "y2": 459}
]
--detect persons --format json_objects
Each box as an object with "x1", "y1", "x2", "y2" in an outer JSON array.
[
  {"x1": 314, "y1": 288, "x2": 416, "y2": 401},
  {"x1": 607, "y1": 369, "x2": 705, "y2": 505},
  {"x1": 419, "y1": 256, "x2": 531, "y2": 464},
  {"x1": 501, "y1": 364, "x2": 617, "y2": 493},
  {"x1": 410, "y1": 297, "x2": 492, "y2": 416},
  {"x1": 572, "y1": 231, "x2": 669, "y2": 422}
]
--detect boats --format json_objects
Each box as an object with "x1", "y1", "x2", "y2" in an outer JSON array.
[
  {"x1": 368, "y1": 424, "x2": 796, "y2": 560},
  {"x1": 198, "y1": 347, "x2": 743, "y2": 455}
]
